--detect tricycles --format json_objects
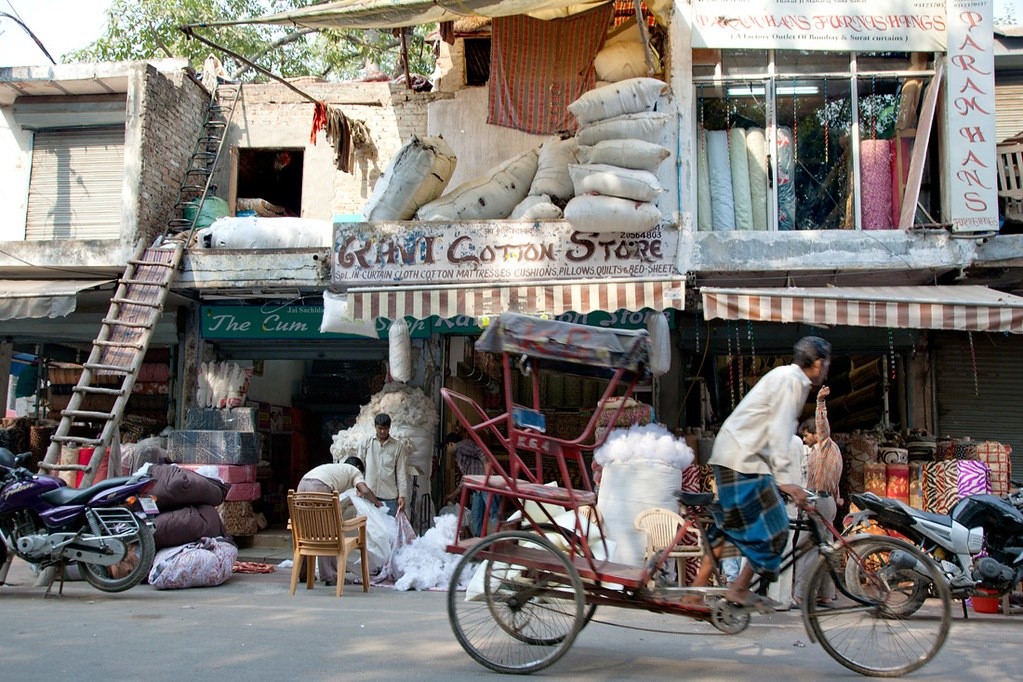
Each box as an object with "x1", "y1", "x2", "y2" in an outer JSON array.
[{"x1": 442, "y1": 312, "x2": 952, "y2": 676}]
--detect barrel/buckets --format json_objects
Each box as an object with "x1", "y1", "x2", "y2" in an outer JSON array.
[{"x1": 970, "y1": 587, "x2": 999, "y2": 614}]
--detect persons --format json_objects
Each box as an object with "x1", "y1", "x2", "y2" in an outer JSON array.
[
  {"x1": 297, "y1": 456, "x2": 383, "y2": 586},
  {"x1": 681, "y1": 337, "x2": 844, "y2": 607},
  {"x1": 362, "y1": 414, "x2": 408, "y2": 576},
  {"x1": 444, "y1": 432, "x2": 501, "y2": 538}
]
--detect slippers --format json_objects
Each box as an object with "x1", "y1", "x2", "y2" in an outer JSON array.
[{"x1": 726, "y1": 592, "x2": 783, "y2": 615}]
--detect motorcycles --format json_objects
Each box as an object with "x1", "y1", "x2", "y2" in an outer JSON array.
[
  {"x1": 1, "y1": 449, "x2": 159, "y2": 599},
  {"x1": 845, "y1": 492, "x2": 1023, "y2": 617}
]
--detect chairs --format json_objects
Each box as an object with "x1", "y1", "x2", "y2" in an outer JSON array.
[
  {"x1": 442, "y1": 387, "x2": 608, "y2": 569},
  {"x1": 997, "y1": 144, "x2": 1023, "y2": 222},
  {"x1": 288, "y1": 488, "x2": 370, "y2": 599},
  {"x1": 633, "y1": 508, "x2": 705, "y2": 588}
]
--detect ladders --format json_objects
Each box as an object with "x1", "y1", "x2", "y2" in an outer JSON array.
[
  {"x1": 0, "y1": 238, "x2": 185, "y2": 588},
  {"x1": 160, "y1": 84, "x2": 241, "y2": 247}
]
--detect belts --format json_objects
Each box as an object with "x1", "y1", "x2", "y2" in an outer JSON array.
[{"x1": 809, "y1": 490, "x2": 831, "y2": 498}]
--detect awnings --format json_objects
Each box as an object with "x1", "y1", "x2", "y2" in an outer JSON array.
[
  {"x1": 346, "y1": 273, "x2": 687, "y2": 323},
  {"x1": 698, "y1": 285, "x2": 1023, "y2": 333}
]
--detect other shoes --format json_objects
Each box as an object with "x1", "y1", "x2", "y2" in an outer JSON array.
[
  {"x1": 817, "y1": 602, "x2": 835, "y2": 614},
  {"x1": 300, "y1": 577, "x2": 317, "y2": 582},
  {"x1": 789, "y1": 601, "x2": 802, "y2": 611},
  {"x1": 325, "y1": 578, "x2": 352, "y2": 586}
]
